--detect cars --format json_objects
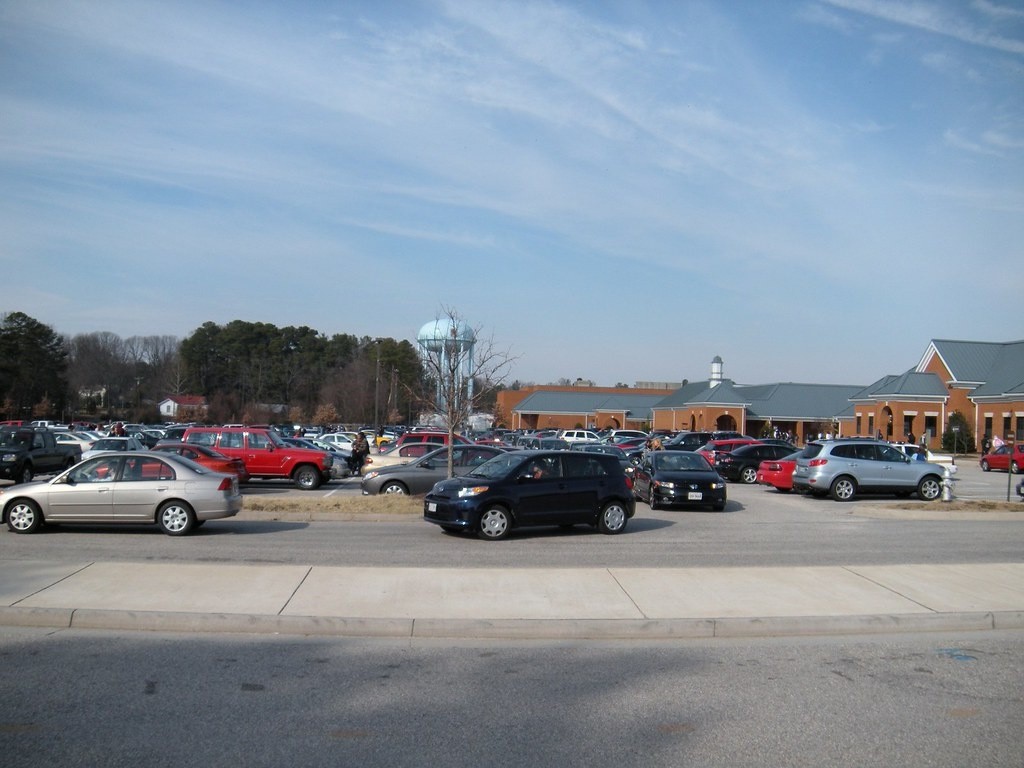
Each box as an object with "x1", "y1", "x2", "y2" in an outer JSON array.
[
  {"x1": 633, "y1": 450, "x2": 727, "y2": 511},
  {"x1": 280, "y1": 437, "x2": 351, "y2": 480},
  {"x1": 474, "y1": 427, "x2": 802, "y2": 483},
  {"x1": 361, "y1": 440, "x2": 468, "y2": 474},
  {"x1": 0, "y1": 450, "x2": 243, "y2": 536},
  {"x1": 32, "y1": 419, "x2": 474, "y2": 445},
  {"x1": 92, "y1": 441, "x2": 245, "y2": 483},
  {"x1": 82, "y1": 437, "x2": 146, "y2": 463},
  {"x1": 52, "y1": 432, "x2": 96, "y2": 452},
  {"x1": 360, "y1": 444, "x2": 516, "y2": 496},
  {"x1": 299, "y1": 438, "x2": 352, "y2": 463},
  {"x1": 757, "y1": 449, "x2": 815, "y2": 494},
  {"x1": 979, "y1": 444, "x2": 1024, "y2": 474},
  {"x1": 862, "y1": 442, "x2": 957, "y2": 476}
]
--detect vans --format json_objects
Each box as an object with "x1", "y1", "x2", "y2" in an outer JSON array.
[{"x1": 423, "y1": 449, "x2": 636, "y2": 541}]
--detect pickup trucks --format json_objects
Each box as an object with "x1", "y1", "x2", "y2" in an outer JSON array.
[{"x1": 0, "y1": 424, "x2": 82, "y2": 484}]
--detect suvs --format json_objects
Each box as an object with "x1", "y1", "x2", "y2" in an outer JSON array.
[
  {"x1": 179, "y1": 426, "x2": 336, "y2": 490},
  {"x1": 156, "y1": 424, "x2": 193, "y2": 445},
  {"x1": 791, "y1": 436, "x2": 947, "y2": 502}
]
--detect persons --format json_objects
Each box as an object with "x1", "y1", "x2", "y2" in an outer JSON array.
[
  {"x1": 68, "y1": 423, "x2": 75, "y2": 430},
  {"x1": 352, "y1": 432, "x2": 371, "y2": 477},
  {"x1": 905, "y1": 430, "x2": 916, "y2": 445},
  {"x1": 775, "y1": 429, "x2": 780, "y2": 439},
  {"x1": 91, "y1": 460, "x2": 120, "y2": 482},
  {"x1": 818, "y1": 430, "x2": 824, "y2": 440},
  {"x1": 653, "y1": 437, "x2": 666, "y2": 452},
  {"x1": 536, "y1": 432, "x2": 543, "y2": 438},
  {"x1": 910, "y1": 443, "x2": 927, "y2": 461},
  {"x1": 825, "y1": 431, "x2": 833, "y2": 440},
  {"x1": 106, "y1": 422, "x2": 128, "y2": 437},
  {"x1": 292, "y1": 429, "x2": 302, "y2": 439},
  {"x1": 531, "y1": 458, "x2": 550, "y2": 479},
  {"x1": 919, "y1": 431, "x2": 928, "y2": 449},
  {"x1": 980, "y1": 433, "x2": 990, "y2": 457},
  {"x1": 94, "y1": 423, "x2": 104, "y2": 432},
  {"x1": 992, "y1": 434, "x2": 1005, "y2": 451},
  {"x1": 788, "y1": 429, "x2": 794, "y2": 445}
]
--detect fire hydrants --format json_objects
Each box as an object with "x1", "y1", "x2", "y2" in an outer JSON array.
[{"x1": 940, "y1": 468, "x2": 955, "y2": 501}]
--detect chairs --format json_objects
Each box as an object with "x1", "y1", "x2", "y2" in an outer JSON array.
[{"x1": 122, "y1": 464, "x2": 133, "y2": 480}]
